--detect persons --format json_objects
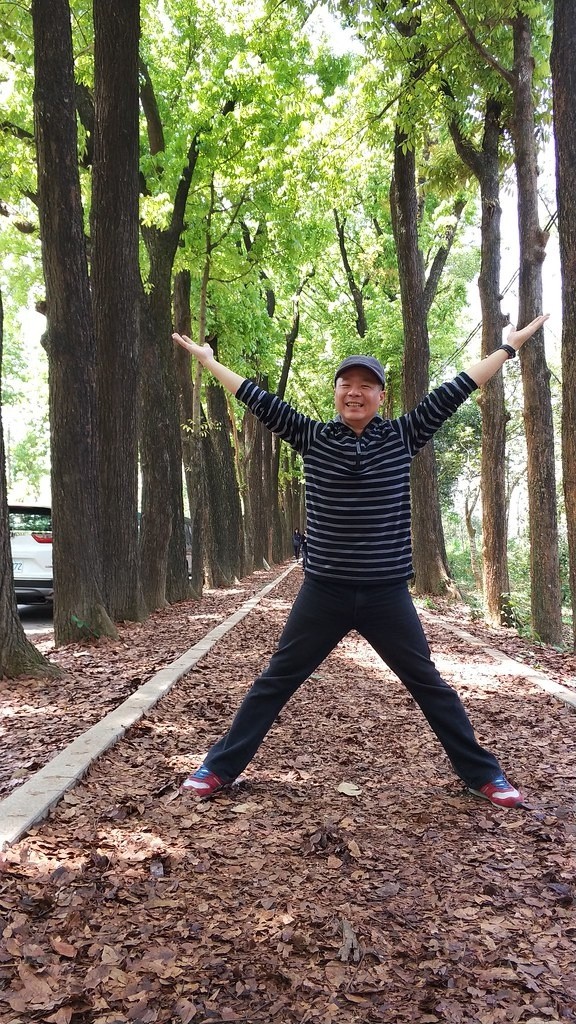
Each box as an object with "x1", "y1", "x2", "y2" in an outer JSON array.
[
  {"x1": 169, "y1": 312, "x2": 551, "y2": 805},
  {"x1": 300, "y1": 529, "x2": 308, "y2": 571},
  {"x1": 292, "y1": 527, "x2": 302, "y2": 559}
]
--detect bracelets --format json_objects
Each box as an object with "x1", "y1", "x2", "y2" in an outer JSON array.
[{"x1": 498, "y1": 344, "x2": 516, "y2": 359}]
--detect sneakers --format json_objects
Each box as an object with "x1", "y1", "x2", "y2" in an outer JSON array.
[
  {"x1": 469, "y1": 774, "x2": 523, "y2": 809},
  {"x1": 180, "y1": 764, "x2": 234, "y2": 798}
]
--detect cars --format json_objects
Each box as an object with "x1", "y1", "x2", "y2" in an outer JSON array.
[{"x1": 7, "y1": 485, "x2": 193, "y2": 612}]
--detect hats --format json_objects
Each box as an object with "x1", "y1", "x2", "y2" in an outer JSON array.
[{"x1": 334, "y1": 355, "x2": 385, "y2": 390}]
